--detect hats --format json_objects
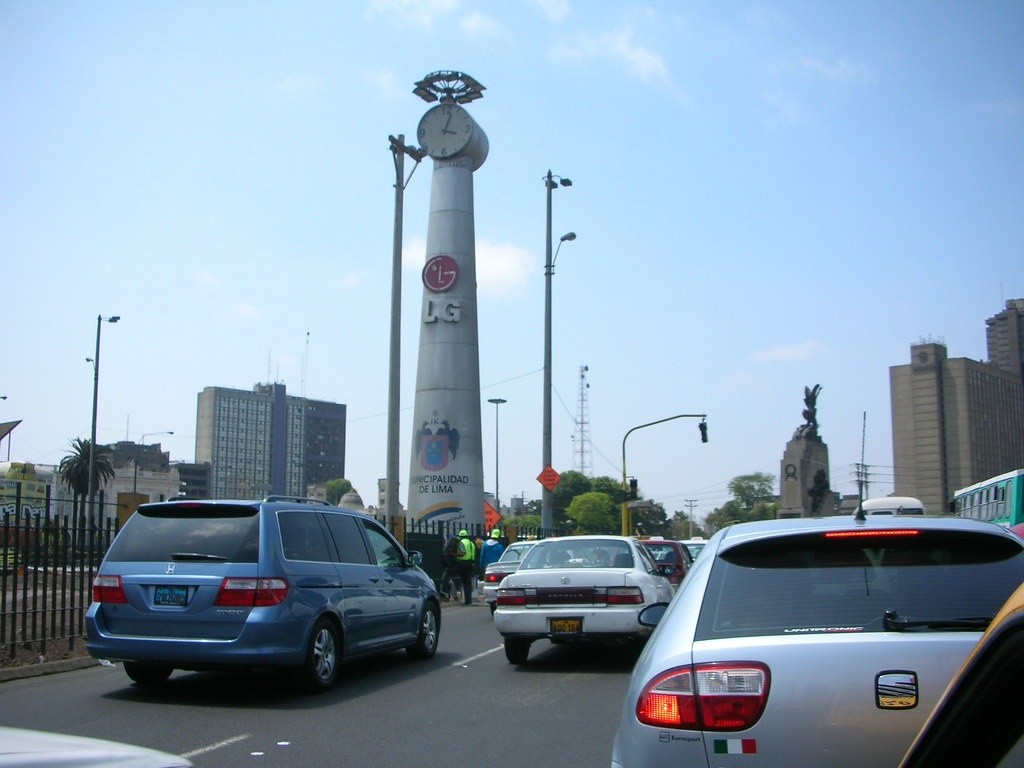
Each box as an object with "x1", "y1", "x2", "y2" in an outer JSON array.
[
  {"x1": 491, "y1": 529, "x2": 500, "y2": 538},
  {"x1": 459, "y1": 530, "x2": 467, "y2": 536}
]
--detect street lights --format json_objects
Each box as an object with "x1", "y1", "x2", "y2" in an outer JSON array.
[
  {"x1": 487, "y1": 397, "x2": 507, "y2": 515},
  {"x1": 83, "y1": 312, "x2": 122, "y2": 548},
  {"x1": 133, "y1": 430, "x2": 175, "y2": 491},
  {"x1": 541, "y1": 167, "x2": 577, "y2": 529}
]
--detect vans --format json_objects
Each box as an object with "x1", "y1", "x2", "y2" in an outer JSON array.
[{"x1": 851, "y1": 496, "x2": 925, "y2": 518}]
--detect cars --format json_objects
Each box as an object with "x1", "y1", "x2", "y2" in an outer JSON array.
[
  {"x1": 895, "y1": 583, "x2": 1024, "y2": 768},
  {"x1": 482, "y1": 535, "x2": 573, "y2": 617},
  {"x1": 611, "y1": 533, "x2": 710, "y2": 593},
  {"x1": 0, "y1": 724, "x2": 198, "y2": 768},
  {"x1": 493, "y1": 534, "x2": 676, "y2": 666}
]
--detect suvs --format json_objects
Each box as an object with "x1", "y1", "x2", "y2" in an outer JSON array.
[
  {"x1": 83, "y1": 493, "x2": 442, "y2": 696},
  {"x1": 611, "y1": 514, "x2": 1024, "y2": 768}
]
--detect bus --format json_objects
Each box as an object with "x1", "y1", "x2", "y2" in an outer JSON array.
[{"x1": 948, "y1": 468, "x2": 1024, "y2": 541}]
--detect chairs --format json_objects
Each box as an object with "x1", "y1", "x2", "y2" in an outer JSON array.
[
  {"x1": 589, "y1": 549, "x2": 611, "y2": 566},
  {"x1": 549, "y1": 551, "x2": 571, "y2": 568}
]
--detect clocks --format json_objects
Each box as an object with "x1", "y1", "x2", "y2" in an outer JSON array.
[{"x1": 419, "y1": 105, "x2": 471, "y2": 156}]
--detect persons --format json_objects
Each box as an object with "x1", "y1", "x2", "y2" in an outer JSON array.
[
  {"x1": 480, "y1": 529, "x2": 504, "y2": 572},
  {"x1": 457, "y1": 530, "x2": 475, "y2": 605}
]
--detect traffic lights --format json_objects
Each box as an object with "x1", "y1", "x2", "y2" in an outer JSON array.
[
  {"x1": 699, "y1": 421, "x2": 709, "y2": 444},
  {"x1": 628, "y1": 479, "x2": 638, "y2": 500}
]
